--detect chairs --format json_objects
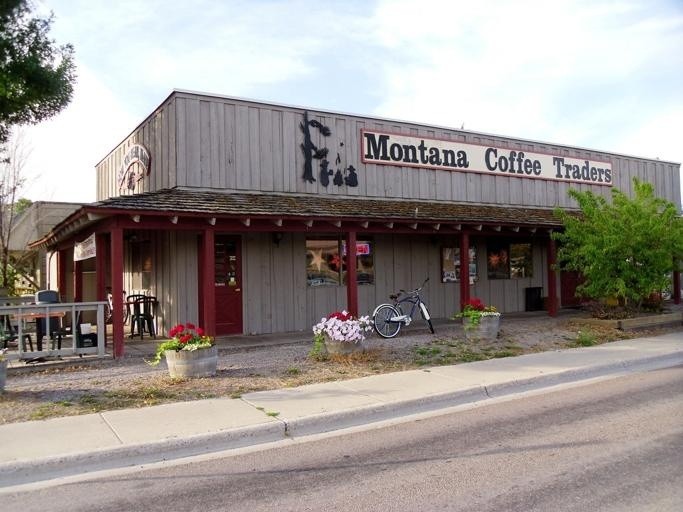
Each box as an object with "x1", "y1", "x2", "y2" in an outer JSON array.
[
  {"x1": 0, "y1": 303, "x2": 33, "y2": 352},
  {"x1": 126, "y1": 294, "x2": 156, "y2": 340},
  {"x1": 52, "y1": 299, "x2": 81, "y2": 351}
]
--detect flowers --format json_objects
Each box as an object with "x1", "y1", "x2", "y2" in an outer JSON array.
[
  {"x1": 309, "y1": 311, "x2": 374, "y2": 357},
  {"x1": 0, "y1": 347, "x2": 8, "y2": 362},
  {"x1": 450, "y1": 297, "x2": 500, "y2": 330},
  {"x1": 143, "y1": 323, "x2": 214, "y2": 366}
]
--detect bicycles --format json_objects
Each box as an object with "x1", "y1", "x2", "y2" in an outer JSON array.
[{"x1": 372, "y1": 275, "x2": 435, "y2": 338}]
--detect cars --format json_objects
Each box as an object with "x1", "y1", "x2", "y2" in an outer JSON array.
[
  {"x1": 306, "y1": 272, "x2": 326, "y2": 286},
  {"x1": 355, "y1": 271, "x2": 373, "y2": 284}
]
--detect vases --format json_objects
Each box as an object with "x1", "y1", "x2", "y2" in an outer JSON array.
[
  {"x1": 165, "y1": 345, "x2": 217, "y2": 378},
  {"x1": 0, "y1": 360, "x2": 8, "y2": 395},
  {"x1": 463, "y1": 316, "x2": 499, "y2": 342},
  {"x1": 324, "y1": 333, "x2": 363, "y2": 354}
]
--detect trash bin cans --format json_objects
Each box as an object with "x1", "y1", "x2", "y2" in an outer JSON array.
[
  {"x1": 525, "y1": 287, "x2": 543, "y2": 312},
  {"x1": 35, "y1": 290, "x2": 61, "y2": 335}
]
